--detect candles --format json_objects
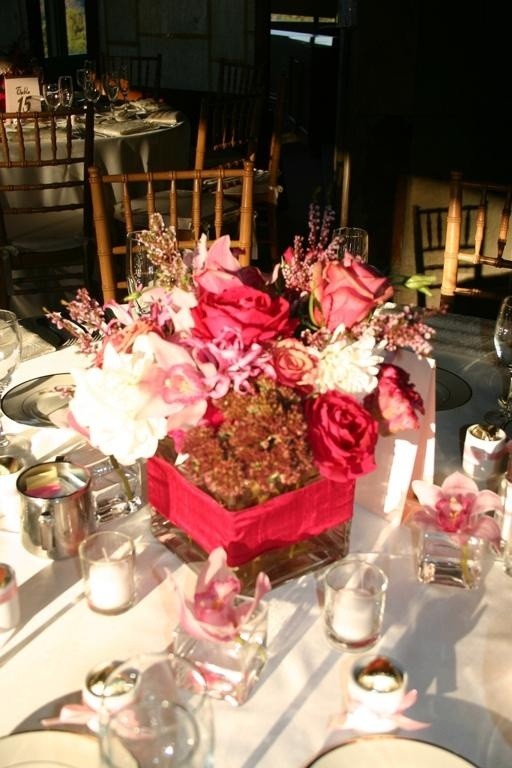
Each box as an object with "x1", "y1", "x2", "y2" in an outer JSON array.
[
  {"x1": 332, "y1": 581, "x2": 377, "y2": 641},
  {"x1": 88, "y1": 544, "x2": 133, "y2": 609}
]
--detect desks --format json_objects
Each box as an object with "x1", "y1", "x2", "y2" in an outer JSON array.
[
  {"x1": 0, "y1": 98, "x2": 191, "y2": 239},
  {"x1": 1, "y1": 347, "x2": 511, "y2": 768}
]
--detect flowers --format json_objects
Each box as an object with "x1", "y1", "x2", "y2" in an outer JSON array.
[
  {"x1": 408, "y1": 471, "x2": 505, "y2": 556},
  {"x1": 42, "y1": 203, "x2": 439, "y2": 509},
  {"x1": 164, "y1": 547, "x2": 273, "y2": 642}
]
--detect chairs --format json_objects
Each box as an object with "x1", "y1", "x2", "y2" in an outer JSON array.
[
  {"x1": 193, "y1": 99, "x2": 286, "y2": 260},
  {"x1": 436, "y1": 167, "x2": 512, "y2": 325},
  {"x1": 86, "y1": 155, "x2": 259, "y2": 308},
  {"x1": 100, "y1": 51, "x2": 165, "y2": 100},
  {"x1": 410, "y1": 199, "x2": 491, "y2": 307},
  {"x1": 1, "y1": 111, "x2": 97, "y2": 307},
  {"x1": 216, "y1": 56, "x2": 273, "y2": 98}
]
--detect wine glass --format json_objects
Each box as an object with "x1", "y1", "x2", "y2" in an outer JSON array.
[
  {"x1": 59, "y1": 76, "x2": 73, "y2": 130},
  {"x1": 0, "y1": 307, "x2": 32, "y2": 457},
  {"x1": 83, "y1": 79, "x2": 102, "y2": 126},
  {"x1": 76, "y1": 69, "x2": 86, "y2": 103},
  {"x1": 82, "y1": 57, "x2": 97, "y2": 86},
  {"x1": 43, "y1": 83, "x2": 60, "y2": 130},
  {"x1": 119, "y1": 71, "x2": 130, "y2": 120},
  {"x1": 483, "y1": 293, "x2": 512, "y2": 439},
  {"x1": 107, "y1": 74, "x2": 119, "y2": 124}
]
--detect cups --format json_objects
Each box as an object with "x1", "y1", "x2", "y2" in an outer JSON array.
[
  {"x1": 412, "y1": 515, "x2": 491, "y2": 592},
  {"x1": 79, "y1": 531, "x2": 140, "y2": 617},
  {"x1": 171, "y1": 590, "x2": 272, "y2": 710},
  {"x1": 346, "y1": 651, "x2": 409, "y2": 712},
  {"x1": 461, "y1": 422, "x2": 507, "y2": 485},
  {"x1": 320, "y1": 558, "x2": 389, "y2": 651},
  {"x1": 82, "y1": 658, "x2": 143, "y2": 709},
  {"x1": 0, "y1": 561, "x2": 22, "y2": 630},
  {"x1": 331, "y1": 227, "x2": 369, "y2": 266},
  {"x1": 123, "y1": 228, "x2": 179, "y2": 297},
  {"x1": 17, "y1": 456, "x2": 99, "y2": 563},
  {"x1": 98, "y1": 650, "x2": 215, "y2": 768},
  {"x1": 91, "y1": 453, "x2": 143, "y2": 524}
]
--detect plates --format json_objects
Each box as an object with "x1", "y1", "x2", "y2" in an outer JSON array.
[
  {"x1": 300, "y1": 736, "x2": 479, "y2": 767},
  {"x1": 435, "y1": 367, "x2": 473, "y2": 413},
  {"x1": 1, "y1": 373, "x2": 80, "y2": 429},
  {"x1": 0, "y1": 729, "x2": 138, "y2": 768}
]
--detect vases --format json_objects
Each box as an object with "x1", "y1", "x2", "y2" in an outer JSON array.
[{"x1": 144, "y1": 453, "x2": 355, "y2": 591}]
